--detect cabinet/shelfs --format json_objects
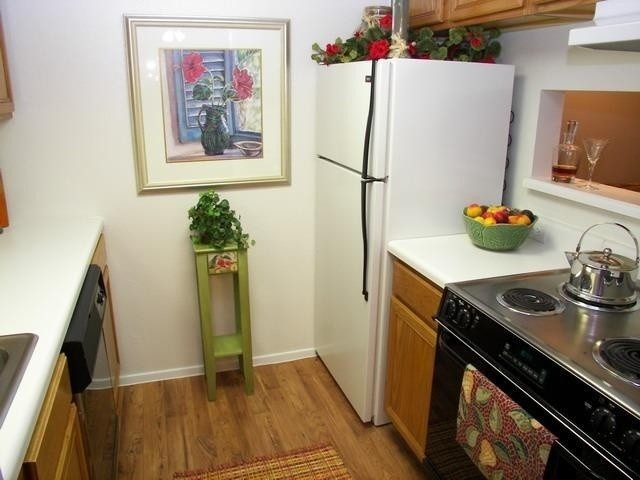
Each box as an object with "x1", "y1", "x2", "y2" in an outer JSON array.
[
  {"x1": 384, "y1": 261, "x2": 443, "y2": 463},
  {"x1": 409, "y1": 1, "x2": 595, "y2": 34},
  {"x1": 90, "y1": 233, "x2": 121, "y2": 413},
  {"x1": 23, "y1": 353, "x2": 89, "y2": 480}
]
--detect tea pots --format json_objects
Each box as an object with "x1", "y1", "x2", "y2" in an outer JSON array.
[{"x1": 564, "y1": 222, "x2": 640, "y2": 307}]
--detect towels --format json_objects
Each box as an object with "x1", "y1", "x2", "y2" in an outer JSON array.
[{"x1": 455, "y1": 364, "x2": 557, "y2": 480}]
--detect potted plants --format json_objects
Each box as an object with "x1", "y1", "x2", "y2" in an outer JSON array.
[{"x1": 188, "y1": 189, "x2": 255, "y2": 249}]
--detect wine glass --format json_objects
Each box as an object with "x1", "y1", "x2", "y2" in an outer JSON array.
[{"x1": 575, "y1": 135, "x2": 613, "y2": 193}]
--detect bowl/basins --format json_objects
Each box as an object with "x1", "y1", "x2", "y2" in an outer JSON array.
[
  {"x1": 462, "y1": 205, "x2": 539, "y2": 252},
  {"x1": 233, "y1": 140, "x2": 262, "y2": 157}
]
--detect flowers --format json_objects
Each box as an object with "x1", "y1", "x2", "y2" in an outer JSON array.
[{"x1": 311, "y1": 14, "x2": 501, "y2": 65}]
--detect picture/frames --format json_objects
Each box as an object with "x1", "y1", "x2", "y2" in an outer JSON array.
[{"x1": 123, "y1": 13, "x2": 291, "y2": 195}]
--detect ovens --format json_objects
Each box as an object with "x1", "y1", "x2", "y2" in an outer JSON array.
[
  {"x1": 423, "y1": 315, "x2": 634, "y2": 480},
  {"x1": 60, "y1": 264, "x2": 120, "y2": 480}
]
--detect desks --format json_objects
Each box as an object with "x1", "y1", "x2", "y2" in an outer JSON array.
[{"x1": 193, "y1": 243, "x2": 254, "y2": 401}]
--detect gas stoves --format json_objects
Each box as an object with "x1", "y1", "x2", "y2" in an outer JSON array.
[{"x1": 437, "y1": 264, "x2": 640, "y2": 480}]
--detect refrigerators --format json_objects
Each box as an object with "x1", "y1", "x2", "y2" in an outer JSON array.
[{"x1": 312, "y1": 59, "x2": 515, "y2": 427}]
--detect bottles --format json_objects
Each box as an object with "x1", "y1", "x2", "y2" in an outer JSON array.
[
  {"x1": 551, "y1": 120, "x2": 583, "y2": 185},
  {"x1": 358, "y1": 6, "x2": 393, "y2": 35}
]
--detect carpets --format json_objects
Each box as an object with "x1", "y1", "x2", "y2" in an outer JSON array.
[{"x1": 173, "y1": 442, "x2": 352, "y2": 480}]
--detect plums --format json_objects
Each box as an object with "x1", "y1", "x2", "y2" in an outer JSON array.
[{"x1": 466, "y1": 204, "x2": 534, "y2": 226}]
